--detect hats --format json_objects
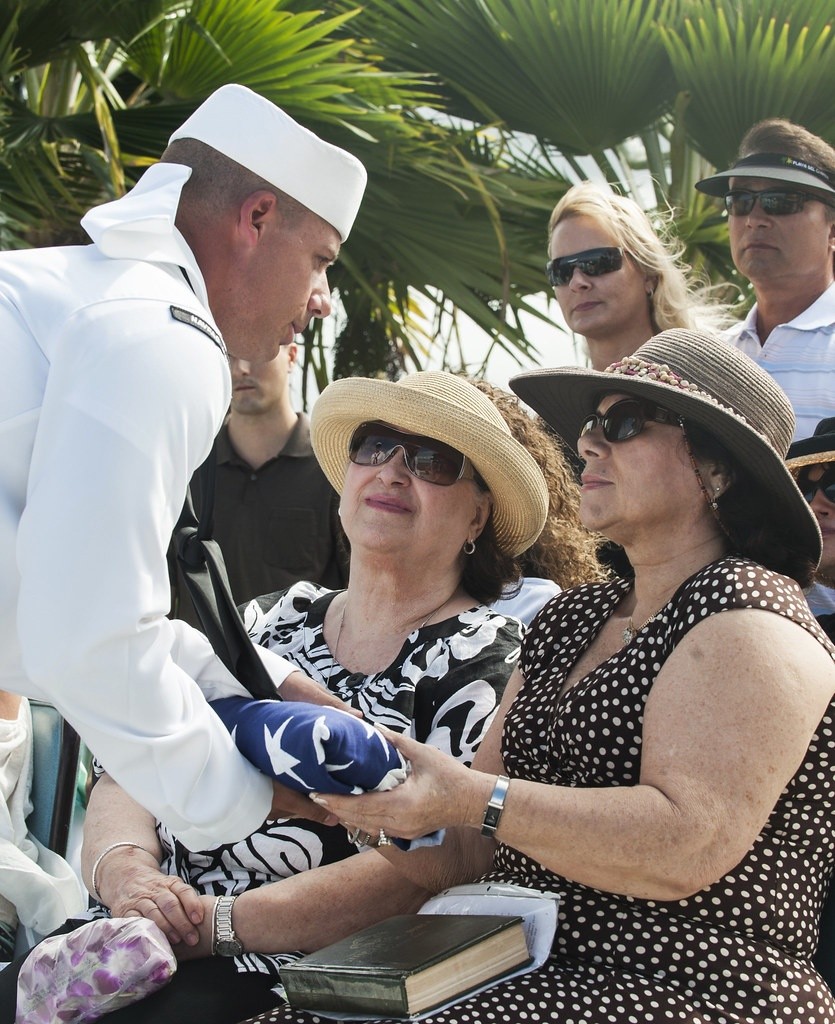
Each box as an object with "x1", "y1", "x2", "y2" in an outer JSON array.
[
  {"x1": 160, "y1": 84, "x2": 367, "y2": 244},
  {"x1": 694, "y1": 154, "x2": 835, "y2": 206},
  {"x1": 509, "y1": 326, "x2": 823, "y2": 588},
  {"x1": 309, "y1": 370, "x2": 550, "y2": 558},
  {"x1": 787, "y1": 417, "x2": 835, "y2": 487}
]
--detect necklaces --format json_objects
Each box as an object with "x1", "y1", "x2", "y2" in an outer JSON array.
[
  {"x1": 326, "y1": 603, "x2": 447, "y2": 699},
  {"x1": 623, "y1": 609, "x2": 663, "y2": 644}
]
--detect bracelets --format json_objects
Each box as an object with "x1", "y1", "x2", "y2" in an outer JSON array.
[
  {"x1": 482, "y1": 774, "x2": 509, "y2": 836},
  {"x1": 347, "y1": 829, "x2": 360, "y2": 843},
  {"x1": 211, "y1": 895, "x2": 222, "y2": 955},
  {"x1": 91, "y1": 842, "x2": 145, "y2": 899},
  {"x1": 361, "y1": 835, "x2": 370, "y2": 847}
]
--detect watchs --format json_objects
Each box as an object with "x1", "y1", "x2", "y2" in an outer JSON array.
[{"x1": 216, "y1": 895, "x2": 242, "y2": 959}]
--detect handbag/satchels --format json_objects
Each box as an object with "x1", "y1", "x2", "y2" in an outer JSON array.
[{"x1": 14, "y1": 917, "x2": 178, "y2": 1024}]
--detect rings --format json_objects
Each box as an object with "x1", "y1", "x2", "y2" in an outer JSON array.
[{"x1": 378, "y1": 828, "x2": 390, "y2": 847}]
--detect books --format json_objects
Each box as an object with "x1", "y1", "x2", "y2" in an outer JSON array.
[{"x1": 279, "y1": 914, "x2": 535, "y2": 1018}]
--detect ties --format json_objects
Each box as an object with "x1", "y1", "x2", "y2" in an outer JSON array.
[{"x1": 176, "y1": 427, "x2": 285, "y2": 704}]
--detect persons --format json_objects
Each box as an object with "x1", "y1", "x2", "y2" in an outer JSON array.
[
  {"x1": 477, "y1": 380, "x2": 604, "y2": 624},
  {"x1": 786, "y1": 416, "x2": 835, "y2": 644},
  {"x1": 545, "y1": 180, "x2": 735, "y2": 373},
  {"x1": 0, "y1": 81, "x2": 366, "y2": 850},
  {"x1": 0, "y1": 688, "x2": 35, "y2": 965},
  {"x1": 166, "y1": 338, "x2": 349, "y2": 626},
  {"x1": 370, "y1": 442, "x2": 386, "y2": 464},
  {"x1": 0, "y1": 371, "x2": 547, "y2": 1024},
  {"x1": 695, "y1": 117, "x2": 835, "y2": 439},
  {"x1": 309, "y1": 327, "x2": 835, "y2": 1024}
]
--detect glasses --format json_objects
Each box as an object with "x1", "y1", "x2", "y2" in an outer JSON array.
[
  {"x1": 796, "y1": 469, "x2": 835, "y2": 506},
  {"x1": 721, "y1": 188, "x2": 835, "y2": 218},
  {"x1": 351, "y1": 421, "x2": 481, "y2": 486},
  {"x1": 579, "y1": 399, "x2": 723, "y2": 442},
  {"x1": 546, "y1": 246, "x2": 629, "y2": 288}
]
